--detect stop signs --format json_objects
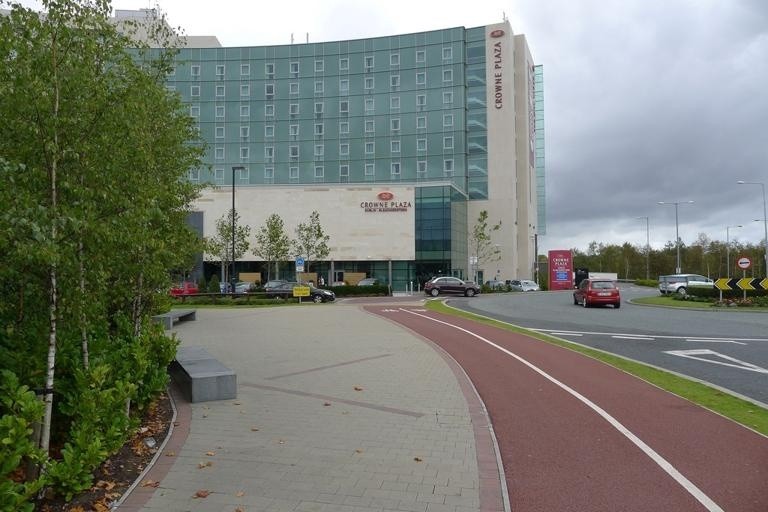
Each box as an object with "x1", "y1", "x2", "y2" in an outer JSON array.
[{"x1": 736, "y1": 256, "x2": 752, "y2": 269}]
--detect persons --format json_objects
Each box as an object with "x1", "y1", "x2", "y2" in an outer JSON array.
[{"x1": 573, "y1": 267, "x2": 587, "y2": 289}]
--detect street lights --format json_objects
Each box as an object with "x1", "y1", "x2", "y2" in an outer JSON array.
[
  {"x1": 231, "y1": 166, "x2": 246, "y2": 284},
  {"x1": 657, "y1": 201, "x2": 696, "y2": 274},
  {"x1": 635, "y1": 216, "x2": 650, "y2": 279},
  {"x1": 737, "y1": 180, "x2": 768, "y2": 279},
  {"x1": 727, "y1": 225, "x2": 742, "y2": 278}
]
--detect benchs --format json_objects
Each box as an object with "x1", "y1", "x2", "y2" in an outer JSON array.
[
  {"x1": 171, "y1": 345, "x2": 237, "y2": 404},
  {"x1": 152, "y1": 309, "x2": 196, "y2": 330}
]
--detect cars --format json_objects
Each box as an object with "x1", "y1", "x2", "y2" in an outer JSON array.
[
  {"x1": 356, "y1": 277, "x2": 379, "y2": 286},
  {"x1": 424, "y1": 277, "x2": 481, "y2": 297},
  {"x1": 510, "y1": 280, "x2": 539, "y2": 292},
  {"x1": 169, "y1": 280, "x2": 350, "y2": 304},
  {"x1": 659, "y1": 273, "x2": 715, "y2": 294},
  {"x1": 573, "y1": 278, "x2": 620, "y2": 309}
]
